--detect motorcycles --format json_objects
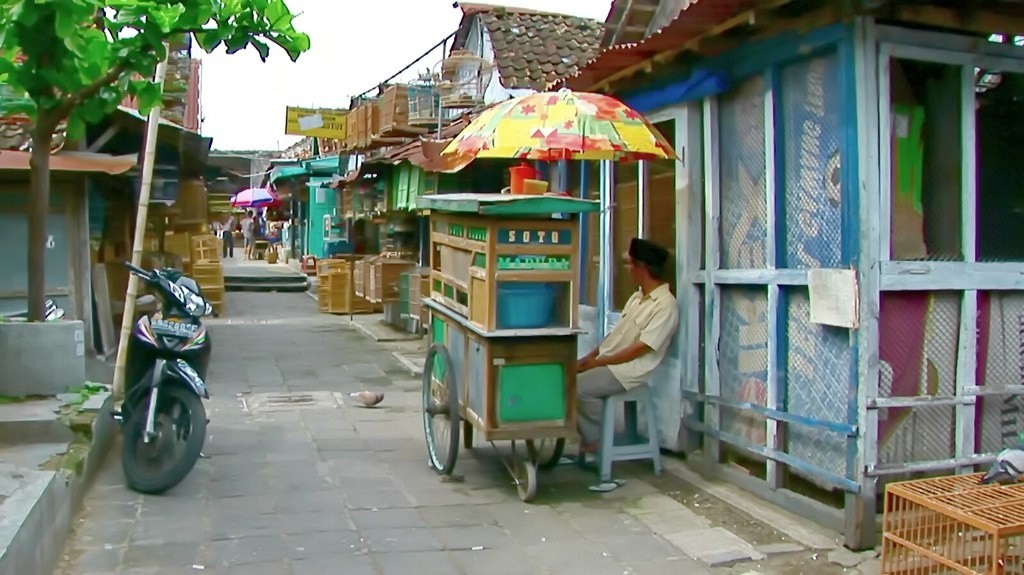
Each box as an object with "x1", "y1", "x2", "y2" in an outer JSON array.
[
  {"x1": 3, "y1": 298, "x2": 65, "y2": 322},
  {"x1": 121, "y1": 261, "x2": 219, "y2": 494}
]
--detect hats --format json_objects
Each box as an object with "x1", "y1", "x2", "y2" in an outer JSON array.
[{"x1": 628, "y1": 237, "x2": 670, "y2": 266}]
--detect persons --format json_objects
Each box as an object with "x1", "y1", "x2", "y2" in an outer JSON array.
[
  {"x1": 223, "y1": 216, "x2": 235, "y2": 258},
  {"x1": 252, "y1": 211, "x2": 265, "y2": 235},
  {"x1": 242, "y1": 210, "x2": 256, "y2": 260},
  {"x1": 562, "y1": 237, "x2": 679, "y2": 456}
]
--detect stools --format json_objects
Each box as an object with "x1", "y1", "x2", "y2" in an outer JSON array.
[
  {"x1": 577, "y1": 382, "x2": 663, "y2": 483},
  {"x1": 301, "y1": 255, "x2": 317, "y2": 276}
]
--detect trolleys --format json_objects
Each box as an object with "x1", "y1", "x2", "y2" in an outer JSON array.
[{"x1": 421, "y1": 343, "x2": 567, "y2": 503}]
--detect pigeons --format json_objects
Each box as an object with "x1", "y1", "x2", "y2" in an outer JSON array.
[{"x1": 347, "y1": 391, "x2": 385, "y2": 408}]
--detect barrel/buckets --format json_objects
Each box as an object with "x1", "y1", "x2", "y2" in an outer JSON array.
[{"x1": 509, "y1": 165, "x2": 534, "y2": 194}]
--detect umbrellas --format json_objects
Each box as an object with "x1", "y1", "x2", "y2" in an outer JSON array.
[
  {"x1": 440, "y1": 88, "x2": 683, "y2": 193},
  {"x1": 231, "y1": 188, "x2": 284, "y2": 223}
]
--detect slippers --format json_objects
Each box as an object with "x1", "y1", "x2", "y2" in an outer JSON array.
[
  {"x1": 557, "y1": 454, "x2": 595, "y2": 464},
  {"x1": 588, "y1": 479, "x2": 627, "y2": 492}
]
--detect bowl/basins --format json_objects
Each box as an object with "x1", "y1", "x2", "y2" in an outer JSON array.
[
  {"x1": 497, "y1": 285, "x2": 557, "y2": 328},
  {"x1": 523, "y1": 179, "x2": 548, "y2": 195}
]
